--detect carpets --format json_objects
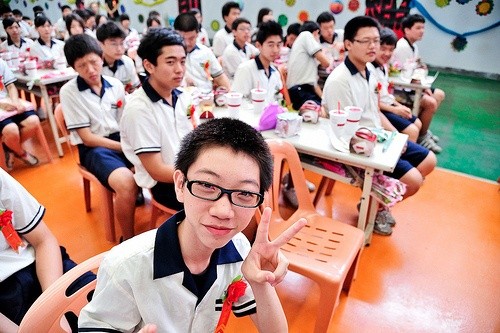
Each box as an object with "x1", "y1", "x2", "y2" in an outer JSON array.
[{"x1": 415, "y1": 73, "x2": 500, "y2": 183}]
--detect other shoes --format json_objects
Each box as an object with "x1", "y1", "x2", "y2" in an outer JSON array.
[
  {"x1": 53, "y1": 102, "x2": 60, "y2": 110},
  {"x1": 119, "y1": 236, "x2": 123, "y2": 244},
  {"x1": 37, "y1": 106, "x2": 47, "y2": 120},
  {"x1": 16, "y1": 152, "x2": 40, "y2": 166},
  {"x1": 4, "y1": 151, "x2": 15, "y2": 168}
]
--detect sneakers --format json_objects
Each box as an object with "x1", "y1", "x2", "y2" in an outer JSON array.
[
  {"x1": 282, "y1": 173, "x2": 316, "y2": 192},
  {"x1": 357, "y1": 202, "x2": 392, "y2": 236},
  {"x1": 427, "y1": 129, "x2": 440, "y2": 143},
  {"x1": 417, "y1": 132, "x2": 442, "y2": 154},
  {"x1": 378, "y1": 206, "x2": 396, "y2": 227},
  {"x1": 283, "y1": 183, "x2": 299, "y2": 207}
]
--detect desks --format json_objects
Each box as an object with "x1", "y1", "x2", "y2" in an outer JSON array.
[
  {"x1": 9, "y1": 65, "x2": 82, "y2": 159},
  {"x1": 315, "y1": 65, "x2": 440, "y2": 118},
  {"x1": 182, "y1": 84, "x2": 411, "y2": 284}
]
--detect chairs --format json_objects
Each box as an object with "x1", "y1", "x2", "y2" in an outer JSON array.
[
  {"x1": 0, "y1": 126, "x2": 53, "y2": 171},
  {"x1": 254, "y1": 141, "x2": 369, "y2": 333},
  {"x1": 143, "y1": 185, "x2": 179, "y2": 226},
  {"x1": 53, "y1": 103, "x2": 117, "y2": 242},
  {"x1": 17, "y1": 252, "x2": 107, "y2": 333}
]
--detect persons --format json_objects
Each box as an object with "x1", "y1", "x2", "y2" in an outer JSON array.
[
  {"x1": 77, "y1": 118, "x2": 307, "y2": 333},
  {"x1": 0, "y1": 0, "x2": 445, "y2": 243},
  {"x1": 0, "y1": 168, "x2": 121, "y2": 333}
]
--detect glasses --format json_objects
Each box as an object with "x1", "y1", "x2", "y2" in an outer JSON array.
[
  {"x1": 354, "y1": 37, "x2": 382, "y2": 46},
  {"x1": 236, "y1": 27, "x2": 251, "y2": 32},
  {"x1": 185, "y1": 177, "x2": 264, "y2": 208}
]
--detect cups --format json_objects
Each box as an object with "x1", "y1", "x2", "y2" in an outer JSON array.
[
  {"x1": 1, "y1": 52, "x2": 12, "y2": 67},
  {"x1": 26, "y1": 61, "x2": 36, "y2": 75},
  {"x1": 329, "y1": 110, "x2": 348, "y2": 136},
  {"x1": 225, "y1": 92, "x2": 242, "y2": 118},
  {"x1": 12, "y1": 51, "x2": 19, "y2": 65},
  {"x1": 251, "y1": 89, "x2": 267, "y2": 113},
  {"x1": 57, "y1": 60, "x2": 67, "y2": 73},
  {"x1": 276, "y1": 113, "x2": 296, "y2": 138},
  {"x1": 345, "y1": 106, "x2": 363, "y2": 131}
]
